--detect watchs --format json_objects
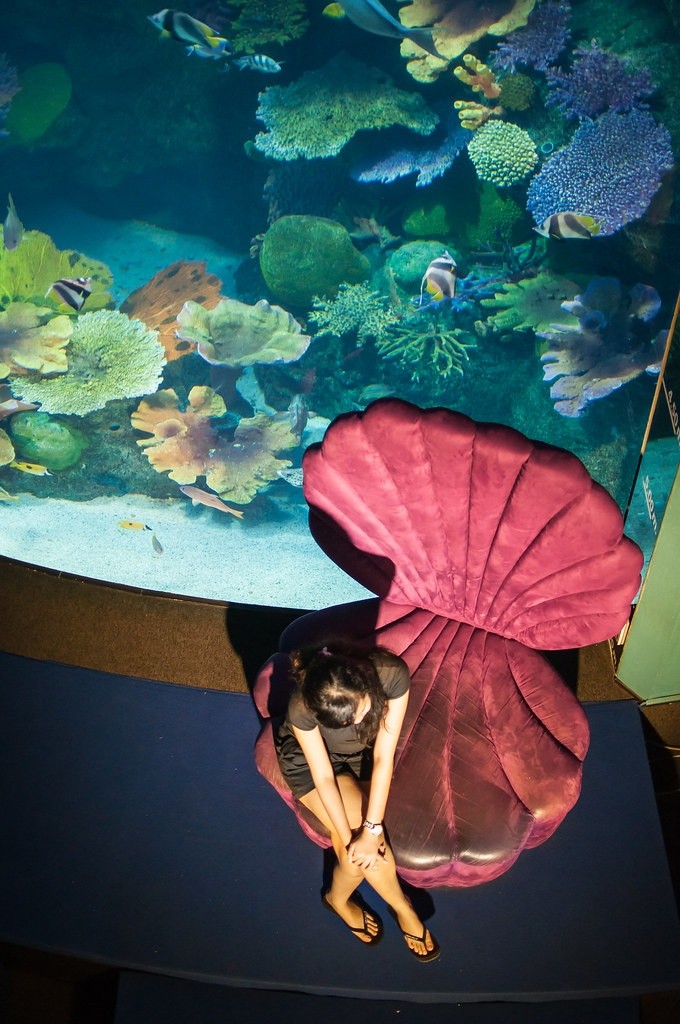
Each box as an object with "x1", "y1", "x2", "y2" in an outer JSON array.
[{"x1": 363, "y1": 820, "x2": 383, "y2": 836}]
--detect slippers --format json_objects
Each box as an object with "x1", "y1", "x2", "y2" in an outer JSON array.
[
  {"x1": 394, "y1": 908, "x2": 441, "y2": 963},
  {"x1": 322, "y1": 892, "x2": 385, "y2": 946}
]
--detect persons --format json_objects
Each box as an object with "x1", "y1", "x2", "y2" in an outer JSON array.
[{"x1": 277, "y1": 642, "x2": 440, "y2": 962}]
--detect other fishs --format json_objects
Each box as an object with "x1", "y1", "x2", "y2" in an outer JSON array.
[
  {"x1": 116, "y1": 520, "x2": 164, "y2": 556},
  {"x1": 531, "y1": 212, "x2": 604, "y2": 239},
  {"x1": 322, "y1": 2, "x2": 345, "y2": 21},
  {"x1": 46, "y1": 277, "x2": 91, "y2": 311},
  {"x1": 3, "y1": 191, "x2": 24, "y2": 251},
  {"x1": 0, "y1": 486, "x2": 19, "y2": 504},
  {"x1": 147, "y1": 9, "x2": 281, "y2": 74},
  {"x1": 179, "y1": 486, "x2": 245, "y2": 519},
  {"x1": 419, "y1": 250, "x2": 458, "y2": 305},
  {"x1": 9, "y1": 460, "x2": 47, "y2": 476},
  {"x1": 277, "y1": 468, "x2": 303, "y2": 488}
]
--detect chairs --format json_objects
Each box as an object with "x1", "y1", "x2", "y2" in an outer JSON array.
[{"x1": 250, "y1": 398, "x2": 644, "y2": 888}]
